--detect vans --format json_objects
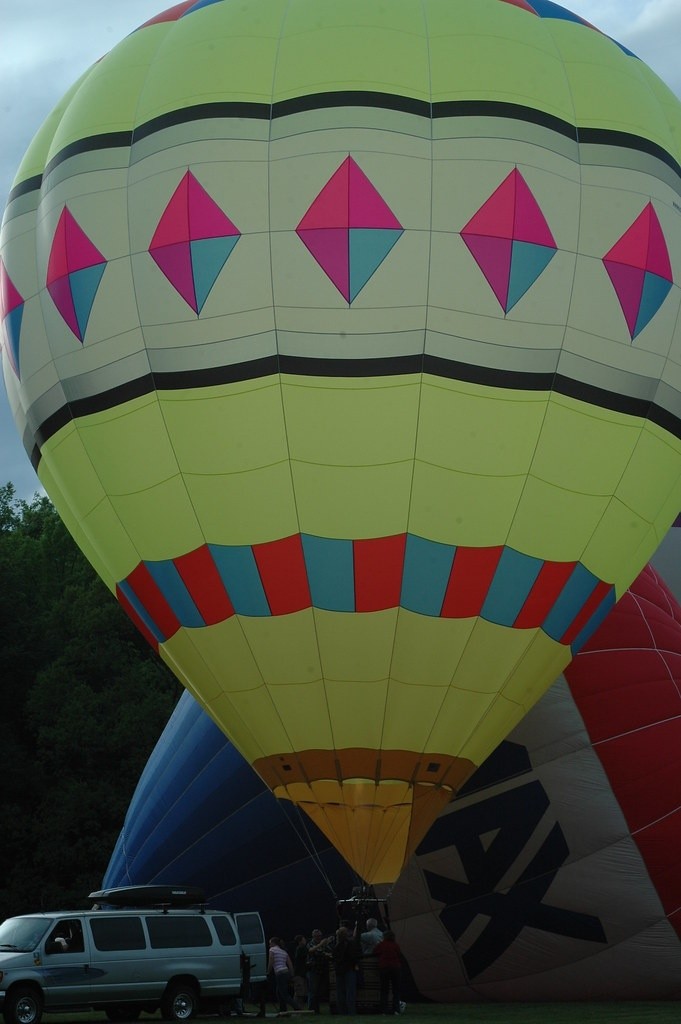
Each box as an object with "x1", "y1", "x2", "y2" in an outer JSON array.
[{"x1": 1, "y1": 881, "x2": 269, "y2": 1024}]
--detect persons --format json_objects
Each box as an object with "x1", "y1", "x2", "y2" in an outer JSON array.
[
  {"x1": 222, "y1": 918, "x2": 359, "y2": 1016},
  {"x1": 360, "y1": 916, "x2": 385, "y2": 1014},
  {"x1": 372, "y1": 930, "x2": 404, "y2": 1016},
  {"x1": 46, "y1": 923, "x2": 74, "y2": 952}
]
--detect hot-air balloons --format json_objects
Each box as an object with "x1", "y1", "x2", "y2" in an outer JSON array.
[
  {"x1": 90, "y1": 559, "x2": 681, "y2": 1005},
  {"x1": 0, "y1": 1, "x2": 681, "y2": 1017}
]
